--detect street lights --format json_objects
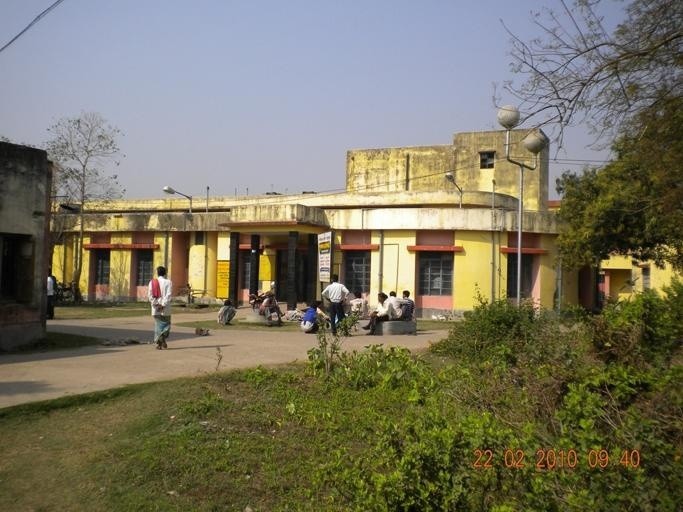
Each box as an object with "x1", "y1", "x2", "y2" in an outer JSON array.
[
  {"x1": 497, "y1": 105, "x2": 549, "y2": 312},
  {"x1": 444, "y1": 172, "x2": 462, "y2": 209}
]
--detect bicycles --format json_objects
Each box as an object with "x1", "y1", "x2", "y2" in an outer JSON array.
[{"x1": 56, "y1": 279, "x2": 76, "y2": 306}]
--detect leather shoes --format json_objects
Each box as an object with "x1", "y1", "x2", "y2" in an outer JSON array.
[{"x1": 362, "y1": 326, "x2": 370, "y2": 329}]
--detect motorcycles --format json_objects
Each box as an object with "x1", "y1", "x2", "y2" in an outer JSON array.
[{"x1": 248, "y1": 280, "x2": 277, "y2": 312}]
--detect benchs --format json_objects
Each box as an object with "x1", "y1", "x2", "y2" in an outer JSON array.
[{"x1": 376, "y1": 321, "x2": 417, "y2": 334}]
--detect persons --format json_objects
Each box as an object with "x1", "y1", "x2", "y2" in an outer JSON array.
[
  {"x1": 259, "y1": 294, "x2": 286, "y2": 327},
  {"x1": 317, "y1": 301, "x2": 331, "y2": 328},
  {"x1": 46, "y1": 267, "x2": 58, "y2": 319},
  {"x1": 217, "y1": 300, "x2": 237, "y2": 325},
  {"x1": 361, "y1": 292, "x2": 393, "y2": 335},
  {"x1": 322, "y1": 274, "x2": 352, "y2": 337},
  {"x1": 399, "y1": 291, "x2": 414, "y2": 321},
  {"x1": 147, "y1": 266, "x2": 173, "y2": 350},
  {"x1": 300, "y1": 302, "x2": 319, "y2": 334},
  {"x1": 388, "y1": 291, "x2": 408, "y2": 320},
  {"x1": 349, "y1": 291, "x2": 367, "y2": 317}
]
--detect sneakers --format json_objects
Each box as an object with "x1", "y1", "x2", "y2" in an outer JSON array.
[{"x1": 157, "y1": 336, "x2": 167, "y2": 349}]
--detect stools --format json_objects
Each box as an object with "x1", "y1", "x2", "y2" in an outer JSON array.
[{"x1": 247, "y1": 315, "x2": 266, "y2": 321}]
